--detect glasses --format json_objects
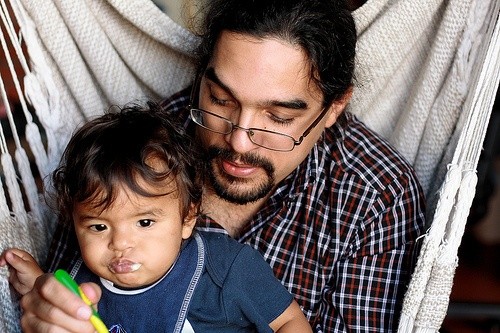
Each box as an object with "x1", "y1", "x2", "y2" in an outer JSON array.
[{"x1": 189, "y1": 71, "x2": 334, "y2": 151}]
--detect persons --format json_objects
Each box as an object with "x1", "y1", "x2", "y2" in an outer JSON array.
[
  {"x1": 0, "y1": 105, "x2": 312, "y2": 333},
  {"x1": 21, "y1": 0, "x2": 423, "y2": 333},
  {"x1": 448, "y1": 142, "x2": 500, "y2": 306}
]
